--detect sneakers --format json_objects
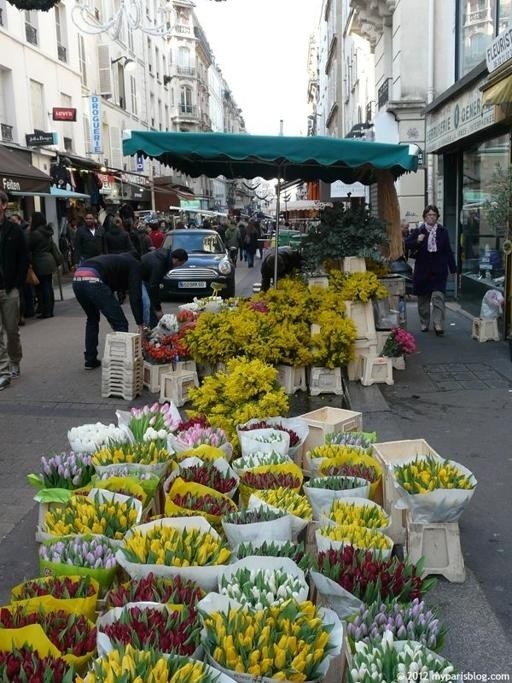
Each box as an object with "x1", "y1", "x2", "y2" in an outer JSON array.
[
  {"x1": 0, "y1": 376, "x2": 11, "y2": 391},
  {"x1": 11, "y1": 364, "x2": 20, "y2": 378},
  {"x1": 84, "y1": 360, "x2": 101, "y2": 370}
]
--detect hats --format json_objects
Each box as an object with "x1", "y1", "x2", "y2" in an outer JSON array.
[
  {"x1": 231, "y1": 220, "x2": 237, "y2": 225},
  {"x1": 113, "y1": 217, "x2": 122, "y2": 225}
]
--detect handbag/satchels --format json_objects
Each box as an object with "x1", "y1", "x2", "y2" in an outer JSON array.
[
  {"x1": 244, "y1": 234, "x2": 250, "y2": 244},
  {"x1": 51, "y1": 242, "x2": 64, "y2": 266}
]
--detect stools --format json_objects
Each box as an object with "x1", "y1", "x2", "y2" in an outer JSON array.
[{"x1": 471, "y1": 316, "x2": 500, "y2": 342}]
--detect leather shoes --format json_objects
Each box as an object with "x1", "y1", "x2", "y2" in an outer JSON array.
[{"x1": 37, "y1": 313, "x2": 53, "y2": 319}]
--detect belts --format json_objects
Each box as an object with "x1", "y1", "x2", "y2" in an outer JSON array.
[{"x1": 73, "y1": 277, "x2": 103, "y2": 283}]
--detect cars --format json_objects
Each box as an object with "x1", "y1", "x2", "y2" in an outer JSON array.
[
  {"x1": 156, "y1": 229, "x2": 234, "y2": 296},
  {"x1": 148, "y1": 208, "x2": 273, "y2": 230}
]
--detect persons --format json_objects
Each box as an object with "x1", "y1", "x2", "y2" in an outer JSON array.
[
  {"x1": 60, "y1": 210, "x2": 306, "y2": 304},
  {"x1": 138, "y1": 246, "x2": 189, "y2": 331},
  {"x1": 0, "y1": 190, "x2": 65, "y2": 390},
  {"x1": 405, "y1": 203, "x2": 457, "y2": 336},
  {"x1": 399, "y1": 219, "x2": 419, "y2": 263},
  {"x1": 70, "y1": 247, "x2": 147, "y2": 371}
]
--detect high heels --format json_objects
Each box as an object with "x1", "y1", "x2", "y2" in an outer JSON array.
[
  {"x1": 421, "y1": 324, "x2": 429, "y2": 332},
  {"x1": 434, "y1": 326, "x2": 444, "y2": 336}
]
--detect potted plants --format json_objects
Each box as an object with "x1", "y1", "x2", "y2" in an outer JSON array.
[{"x1": 299, "y1": 202, "x2": 393, "y2": 276}]
